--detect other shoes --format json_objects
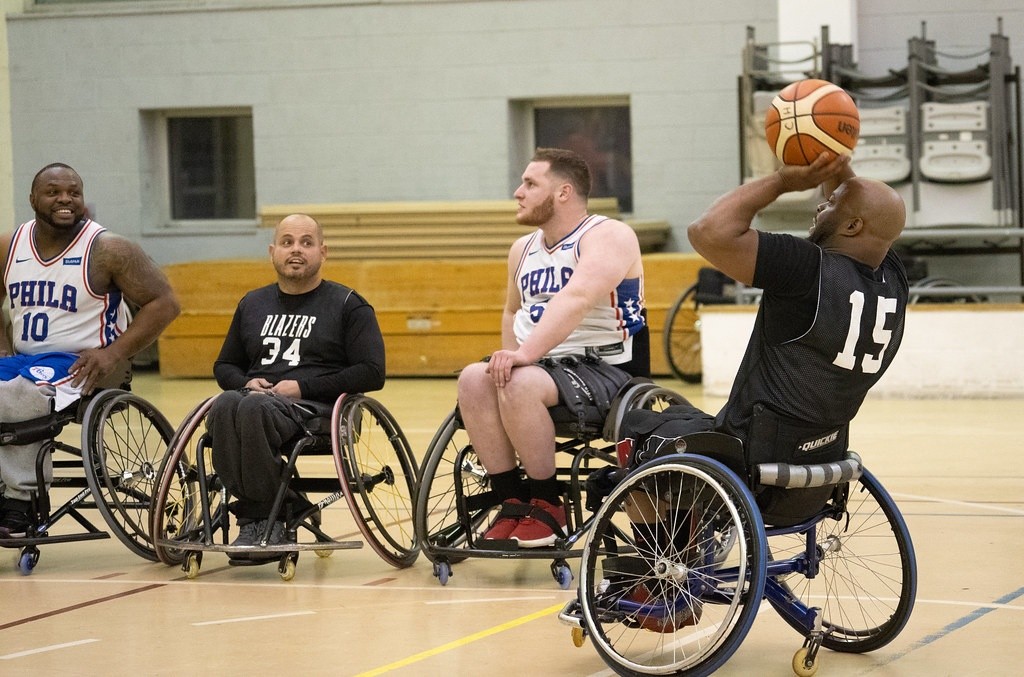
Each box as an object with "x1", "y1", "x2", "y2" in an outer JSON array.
[
  {"x1": 597, "y1": 579, "x2": 681, "y2": 632},
  {"x1": 681, "y1": 597, "x2": 703, "y2": 625}
]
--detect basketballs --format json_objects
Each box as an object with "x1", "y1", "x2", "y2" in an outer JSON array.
[{"x1": 762, "y1": 76, "x2": 863, "y2": 168}]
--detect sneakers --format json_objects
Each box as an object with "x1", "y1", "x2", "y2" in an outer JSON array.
[
  {"x1": 484, "y1": 498, "x2": 525, "y2": 540},
  {"x1": 249, "y1": 519, "x2": 286, "y2": 559},
  {"x1": 225, "y1": 518, "x2": 259, "y2": 558},
  {"x1": 0, "y1": 491, "x2": 51, "y2": 538},
  {"x1": 508, "y1": 498, "x2": 569, "y2": 547}
]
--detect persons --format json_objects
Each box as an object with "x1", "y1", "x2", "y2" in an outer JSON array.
[
  {"x1": 205, "y1": 214, "x2": 385, "y2": 561},
  {"x1": 456, "y1": 148, "x2": 649, "y2": 548},
  {"x1": 595, "y1": 151, "x2": 909, "y2": 636},
  {"x1": 0, "y1": 163, "x2": 181, "y2": 539}
]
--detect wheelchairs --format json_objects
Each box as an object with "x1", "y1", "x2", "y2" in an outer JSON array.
[
  {"x1": 0, "y1": 380, "x2": 198, "y2": 579},
  {"x1": 414, "y1": 306, "x2": 699, "y2": 590},
  {"x1": 557, "y1": 403, "x2": 919, "y2": 677},
  {"x1": 149, "y1": 390, "x2": 422, "y2": 581},
  {"x1": 662, "y1": 238, "x2": 989, "y2": 386}
]
